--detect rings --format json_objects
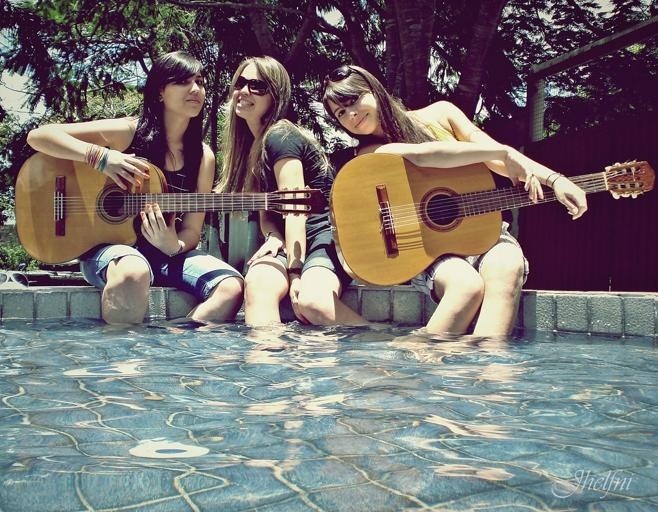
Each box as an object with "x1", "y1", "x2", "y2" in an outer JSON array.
[{"x1": 528, "y1": 174, "x2": 534, "y2": 177}]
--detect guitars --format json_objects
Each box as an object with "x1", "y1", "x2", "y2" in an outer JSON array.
[
  {"x1": 15, "y1": 151, "x2": 329, "y2": 264},
  {"x1": 331, "y1": 153, "x2": 655, "y2": 289}
]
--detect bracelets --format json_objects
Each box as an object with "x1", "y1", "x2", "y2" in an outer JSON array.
[
  {"x1": 84, "y1": 144, "x2": 110, "y2": 174},
  {"x1": 169, "y1": 241, "x2": 185, "y2": 257},
  {"x1": 286, "y1": 268, "x2": 302, "y2": 275},
  {"x1": 551, "y1": 174, "x2": 563, "y2": 188},
  {"x1": 545, "y1": 172, "x2": 560, "y2": 186},
  {"x1": 264, "y1": 231, "x2": 275, "y2": 241}
]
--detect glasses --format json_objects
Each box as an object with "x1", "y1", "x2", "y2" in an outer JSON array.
[
  {"x1": 323, "y1": 66, "x2": 359, "y2": 93},
  {"x1": 234, "y1": 76, "x2": 270, "y2": 96}
]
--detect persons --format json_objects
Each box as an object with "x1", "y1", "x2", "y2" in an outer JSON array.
[
  {"x1": 211, "y1": 53, "x2": 372, "y2": 333},
  {"x1": 24, "y1": 50, "x2": 245, "y2": 330},
  {"x1": 317, "y1": 65, "x2": 592, "y2": 362}
]
